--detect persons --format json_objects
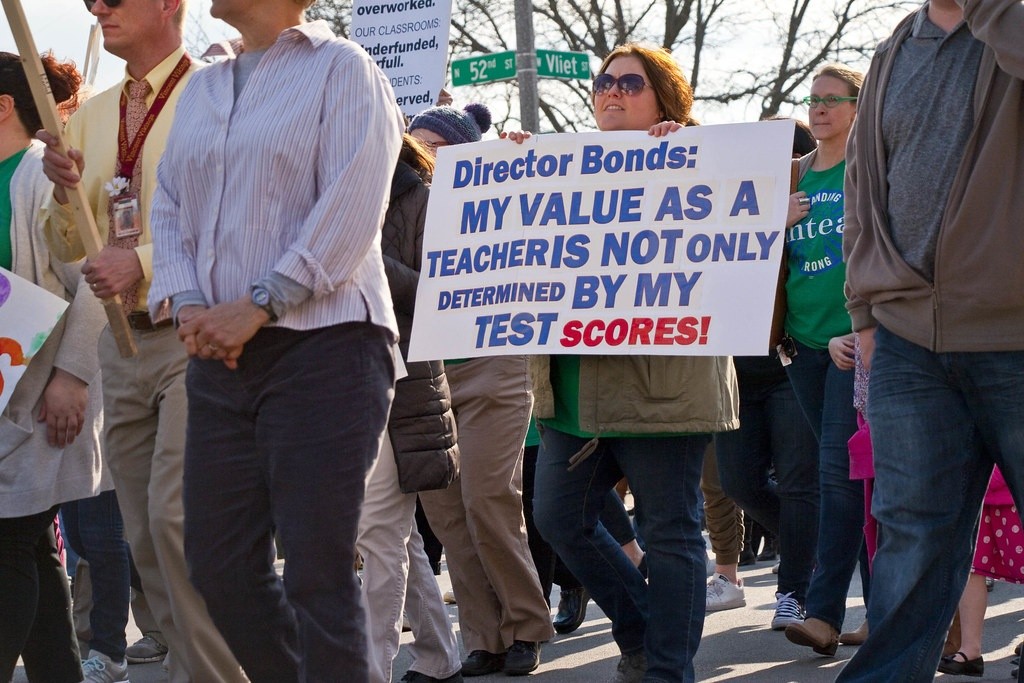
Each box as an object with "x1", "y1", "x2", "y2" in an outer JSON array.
[
  {"x1": 121, "y1": 210, "x2": 133, "y2": 230},
  {"x1": 0, "y1": 0, "x2": 1024, "y2": 683}
]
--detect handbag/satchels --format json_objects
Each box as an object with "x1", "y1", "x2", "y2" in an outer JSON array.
[{"x1": 770, "y1": 158, "x2": 800, "y2": 350}]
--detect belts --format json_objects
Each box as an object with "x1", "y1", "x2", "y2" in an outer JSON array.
[{"x1": 128, "y1": 312, "x2": 173, "y2": 331}]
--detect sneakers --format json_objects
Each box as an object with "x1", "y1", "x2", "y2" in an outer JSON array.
[
  {"x1": 938, "y1": 613, "x2": 963, "y2": 657},
  {"x1": 502, "y1": 640, "x2": 542, "y2": 674},
  {"x1": 839, "y1": 617, "x2": 870, "y2": 645},
  {"x1": 704, "y1": 573, "x2": 746, "y2": 611},
  {"x1": 784, "y1": 617, "x2": 839, "y2": 656},
  {"x1": 461, "y1": 650, "x2": 507, "y2": 677},
  {"x1": 79, "y1": 649, "x2": 129, "y2": 683},
  {"x1": 771, "y1": 590, "x2": 808, "y2": 630},
  {"x1": 125, "y1": 636, "x2": 168, "y2": 662}
]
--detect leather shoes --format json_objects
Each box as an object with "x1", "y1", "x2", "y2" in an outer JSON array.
[{"x1": 551, "y1": 585, "x2": 591, "y2": 635}]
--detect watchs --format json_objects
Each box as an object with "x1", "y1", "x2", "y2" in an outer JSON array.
[{"x1": 252, "y1": 287, "x2": 278, "y2": 322}]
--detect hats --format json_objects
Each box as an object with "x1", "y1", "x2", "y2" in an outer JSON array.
[{"x1": 407, "y1": 103, "x2": 491, "y2": 145}]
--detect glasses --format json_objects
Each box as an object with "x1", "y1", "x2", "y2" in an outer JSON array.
[
  {"x1": 592, "y1": 74, "x2": 653, "y2": 97},
  {"x1": 804, "y1": 96, "x2": 858, "y2": 109},
  {"x1": 83, "y1": 0, "x2": 122, "y2": 11},
  {"x1": 425, "y1": 140, "x2": 448, "y2": 151}
]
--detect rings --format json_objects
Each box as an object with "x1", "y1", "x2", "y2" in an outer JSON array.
[
  {"x1": 209, "y1": 345, "x2": 218, "y2": 350},
  {"x1": 93, "y1": 284, "x2": 97, "y2": 289}
]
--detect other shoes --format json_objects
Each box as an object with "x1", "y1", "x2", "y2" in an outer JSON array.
[
  {"x1": 637, "y1": 551, "x2": 648, "y2": 580},
  {"x1": 403, "y1": 609, "x2": 412, "y2": 631},
  {"x1": 737, "y1": 547, "x2": 755, "y2": 566},
  {"x1": 606, "y1": 650, "x2": 648, "y2": 683},
  {"x1": 937, "y1": 652, "x2": 984, "y2": 677},
  {"x1": 400, "y1": 669, "x2": 464, "y2": 683},
  {"x1": 772, "y1": 561, "x2": 779, "y2": 574},
  {"x1": 758, "y1": 540, "x2": 780, "y2": 561},
  {"x1": 443, "y1": 587, "x2": 456, "y2": 603}
]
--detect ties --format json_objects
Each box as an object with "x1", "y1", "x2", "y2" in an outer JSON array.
[{"x1": 107, "y1": 80, "x2": 152, "y2": 318}]
